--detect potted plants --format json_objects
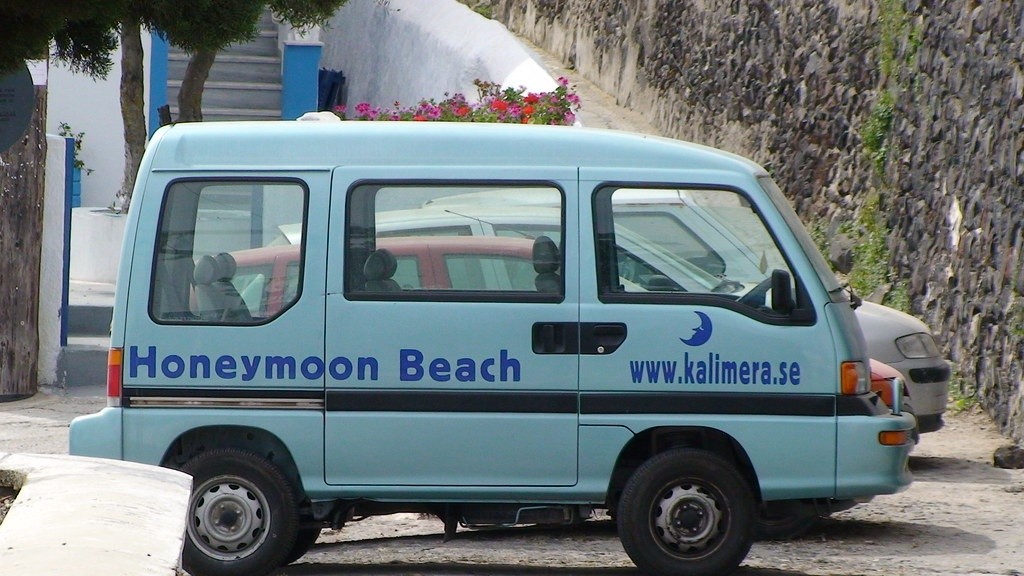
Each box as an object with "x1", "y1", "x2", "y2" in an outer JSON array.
[{"x1": 57, "y1": 121, "x2": 95, "y2": 208}]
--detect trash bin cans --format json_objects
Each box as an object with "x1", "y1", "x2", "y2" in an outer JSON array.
[{"x1": 281, "y1": 39, "x2": 324, "y2": 120}]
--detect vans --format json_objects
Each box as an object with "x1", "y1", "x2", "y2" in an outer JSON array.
[{"x1": 68, "y1": 122, "x2": 918, "y2": 576}]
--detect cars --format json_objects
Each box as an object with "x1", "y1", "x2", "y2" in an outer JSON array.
[{"x1": 190, "y1": 188, "x2": 952, "y2": 542}]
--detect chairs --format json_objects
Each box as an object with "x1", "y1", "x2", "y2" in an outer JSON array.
[
  {"x1": 532, "y1": 235, "x2": 561, "y2": 293},
  {"x1": 362, "y1": 247, "x2": 404, "y2": 290},
  {"x1": 192, "y1": 255, "x2": 237, "y2": 321},
  {"x1": 212, "y1": 252, "x2": 253, "y2": 321}
]
